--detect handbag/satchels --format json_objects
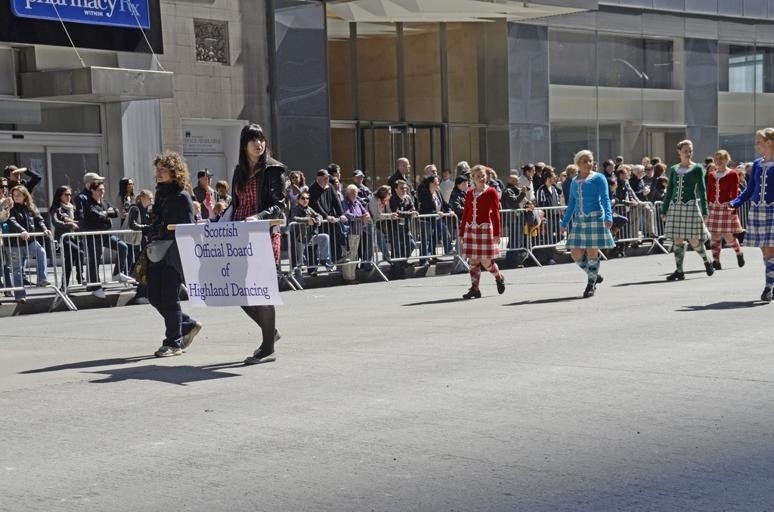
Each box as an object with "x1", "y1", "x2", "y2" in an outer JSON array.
[
  {"x1": 119, "y1": 206, "x2": 143, "y2": 246},
  {"x1": 133, "y1": 242, "x2": 151, "y2": 287}
]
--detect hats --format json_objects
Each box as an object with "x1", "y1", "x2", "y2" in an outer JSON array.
[
  {"x1": 353, "y1": 170, "x2": 365, "y2": 178},
  {"x1": 198, "y1": 170, "x2": 213, "y2": 179},
  {"x1": 455, "y1": 176, "x2": 467, "y2": 185},
  {"x1": 83, "y1": 173, "x2": 105, "y2": 185},
  {"x1": 317, "y1": 168, "x2": 331, "y2": 177}
]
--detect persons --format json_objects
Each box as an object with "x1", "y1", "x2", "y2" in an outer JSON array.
[
  {"x1": 187, "y1": 170, "x2": 231, "y2": 223},
  {"x1": 592, "y1": 155, "x2": 669, "y2": 257},
  {"x1": 222, "y1": 122, "x2": 287, "y2": 365},
  {"x1": 0, "y1": 165, "x2": 52, "y2": 297},
  {"x1": 703, "y1": 149, "x2": 744, "y2": 270},
  {"x1": 658, "y1": 139, "x2": 714, "y2": 281},
  {"x1": 726, "y1": 127, "x2": 774, "y2": 301},
  {"x1": 702, "y1": 158, "x2": 716, "y2": 249},
  {"x1": 117, "y1": 178, "x2": 137, "y2": 228},
  {"x1": 50, "y1": 187, "x2": 87, "y2": 291},
  {"x1": 75, "y1": 172, "x2": 105, "y2": 279},
  {"x1": 727, "y1": 161, "x2": 753, "y2": 246},
  {"x1": 83, "y1": 180, "x2": 133, "y2": 297},
  {"x1": 486, "y1": 162, "x2": 577, "y2": 268},
  {"x1": 416, "y1": 161, "x2": 471, "y2": 265},
  {"x1": 289, "y1": 159, "x2": 419, "y2": 284},
  {"x1": 459, "y1": 165, "x2": 505, "y2": 298},
  {"x1": 128, "y1": 150, "x2": 202, "y2": 358},
  {"x1": 122, "y1": 190, "x2": 153, "y2": 275},
  {"x1": 560, "y1": 149, "x2": 614, "y2": 299}
]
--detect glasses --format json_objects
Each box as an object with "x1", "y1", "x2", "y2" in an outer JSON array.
[
  {"x1": 0, "y1": 185, "x2": 8, "y2": 188},
  {"x1": 300, "y1": 197, "x2": 310, "y2": 200}
]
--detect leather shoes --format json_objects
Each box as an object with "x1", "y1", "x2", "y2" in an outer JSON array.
[
  {"x1": 272, "y1": 329, "x2": 282, "y2": 341},
  {"x1": 245, "y1": 347, "x2": 276, "y2": 365}
]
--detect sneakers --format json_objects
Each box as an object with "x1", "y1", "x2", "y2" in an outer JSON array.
[
  {"x1": 92, "y1": 288, "x2": 106, "y2": 298},
  {"x1": 761, "y1": 288, "x2": 773, "y2": 302},
  {"x1": 180, "y1": 321, "x2": 202, "y2": 350},
  {"x1": 462, "y1": 288, "x2": 481, "y2": 299},
  {"x1": 705, "y1": 262, "x2": 714, "y2": 276},
  {"x1": 737, "y1": 252, "x2": 745, "y2": 267},
  {"x1": 712, "y1": 261, "x2": 721, "y2": 270},
  {"x1": 112, "y1": 272, "x2": 133, "y2": 283},
  {"x1": 496, "y1": 274, "x2": 505, "y2": 294},
  {"x1": 583, "y1": 284, "x2": 596, "y2": 298},
  {"x1": 447, "y1": 249, "x2": 457, "y2": 255},
  {"x1": 155, "y1": 345, "x2": 182, "y2": 357},
  {"x1": 321, "y1": 257, "x2": 333, "y2": 267},
  {"x1": 597, "y1": 275, "x2": 603, "y2": 284},
  {"x1": 4, "y1": 279, "x2": 51, "y2": 302},
  {"x1": 667, "y1": 269, "x2": 685, "y2": 281}
]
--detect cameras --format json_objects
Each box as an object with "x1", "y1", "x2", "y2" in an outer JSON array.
[{"x1": 526, "y1": 186, "x2": 530, "y2": 191}]
